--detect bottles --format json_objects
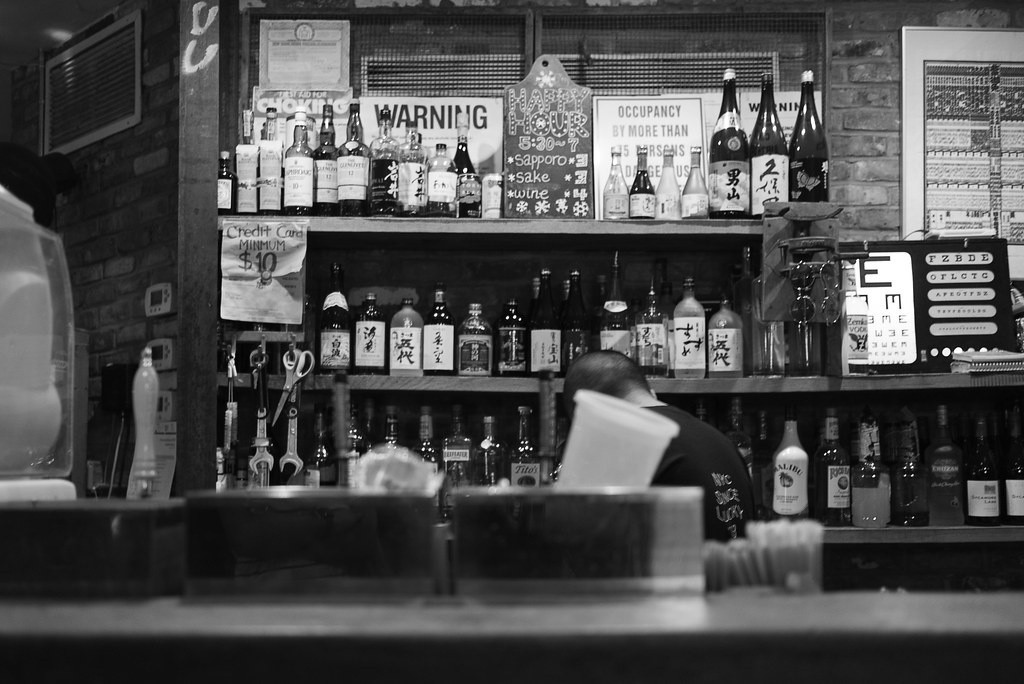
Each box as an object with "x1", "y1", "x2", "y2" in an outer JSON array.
[
  {"x1": 602, "y1": 146, "x2": 629, "y2": 219},
  {"x1": 692, "y1": 394, "x2": 1024, "y2": 529},
  {"x1": 386, "y1": 297, "x2": 424, "y2": 378},
  {"x1": 496, "y1": 298, "x2": 527, "y2": 377},
  {"x1": 422, "y1": 281, "x2": 457, "y2": 375},
  {"x1": 749, "y1": 74, "x2": 789, "y2": 219},
  {"x1": 654, "y1": 148, "x2": 681, "y2": 220},
  {"x1": 680, "y1": 146, "x2": 708, "y2": 218},
  {"x1": 455, "y1": 302, "x2": 494, "y2": 376},
  {"x1": 592, "y1": 250, "x2": 821, "y2": 380},
  {"x1": 788, "y1": 70, "x2": 829, "y2": 202},
  {"x1": 527, "y1": 266, "x2": 590, "y2": 378},
  {"x1": 841, "y1": 296, "x2": 869, "y2": 378},
  {"x1": 352, "y1": 292, "x2": 388, "y2": 374},
  {"x1": 216, "y1": 403, "x2": 540, "y2": 524},
  {"x1": 313, "y1": 261, "x2": 352, "y2": 376},
  {"x1": 629, "y1": 145, "x2": 655, "y2": 219},
  {"x1": 216, "y1": 98, "x2": 502, "y2": 218},
  {"x1": 707, "y1": 68, "x2": 749, "y2": 218}
]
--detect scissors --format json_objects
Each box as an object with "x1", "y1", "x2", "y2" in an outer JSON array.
[{"x1": 271, "y1": 348, "x2": 316, "y2": 427}]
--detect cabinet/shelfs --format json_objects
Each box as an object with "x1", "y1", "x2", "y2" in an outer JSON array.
[{"x1": 216, "y1": 213, "x2": 1024, "y2": 544}]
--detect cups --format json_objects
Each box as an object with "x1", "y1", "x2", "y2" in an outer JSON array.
[{"x1": 554, "y1": 388, "x2": 680, "y2": 485}]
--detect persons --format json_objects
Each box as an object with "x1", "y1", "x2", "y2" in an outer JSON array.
[{"x1": 563, "y1": 349, "x2": 753, "y2": 544}]
